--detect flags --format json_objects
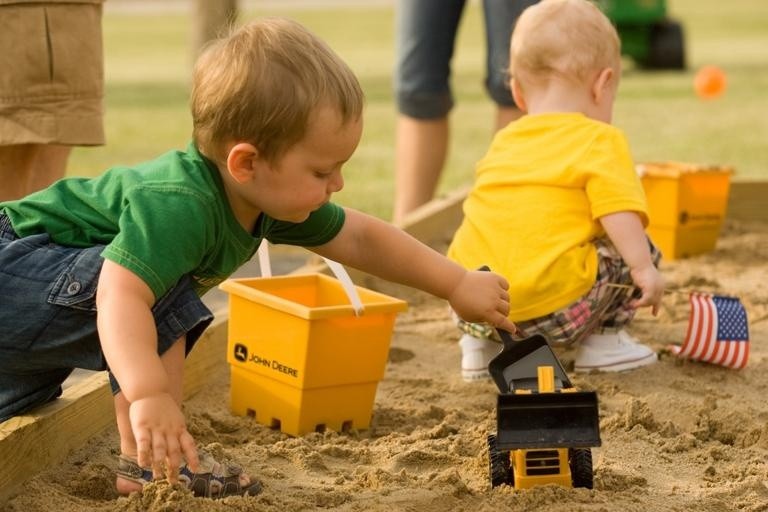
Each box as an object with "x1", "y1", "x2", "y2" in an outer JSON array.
[{"x1": 670, "y1": 292, "x2": 750, "y2": 370}]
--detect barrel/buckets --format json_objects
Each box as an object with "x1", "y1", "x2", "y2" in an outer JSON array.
[
  {"x1": 630, "y1": 157, "x2": 737, "y2": 264},
  {"x1": 218, "y1": 238, "x2": 409, "y2": 440}
]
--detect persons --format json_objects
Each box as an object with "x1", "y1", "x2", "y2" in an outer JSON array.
[
  {"x1": 0, "y1": 0, "x2": 107, "y2": 202},
  {"x1": 391, "y1": 0, "x2": 538, "y2": 222},
  {"x1": 0, "y1": 18, "x2": 518, "y2": 500},
  {"x1": 445, "y1": 0, "x2": 664, "y2": 381}
]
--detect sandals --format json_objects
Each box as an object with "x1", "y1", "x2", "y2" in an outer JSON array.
[{"x1": 114, "y1": 454, "x2": 262, "y2": 504}]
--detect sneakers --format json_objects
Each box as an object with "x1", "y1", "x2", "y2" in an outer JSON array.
[
  {"x1": 573, "y1": 329, "x2": 660, "y2": 376},
  {"x1": 461, "y1": 342, "x2": 502, "y2": 381}
]
